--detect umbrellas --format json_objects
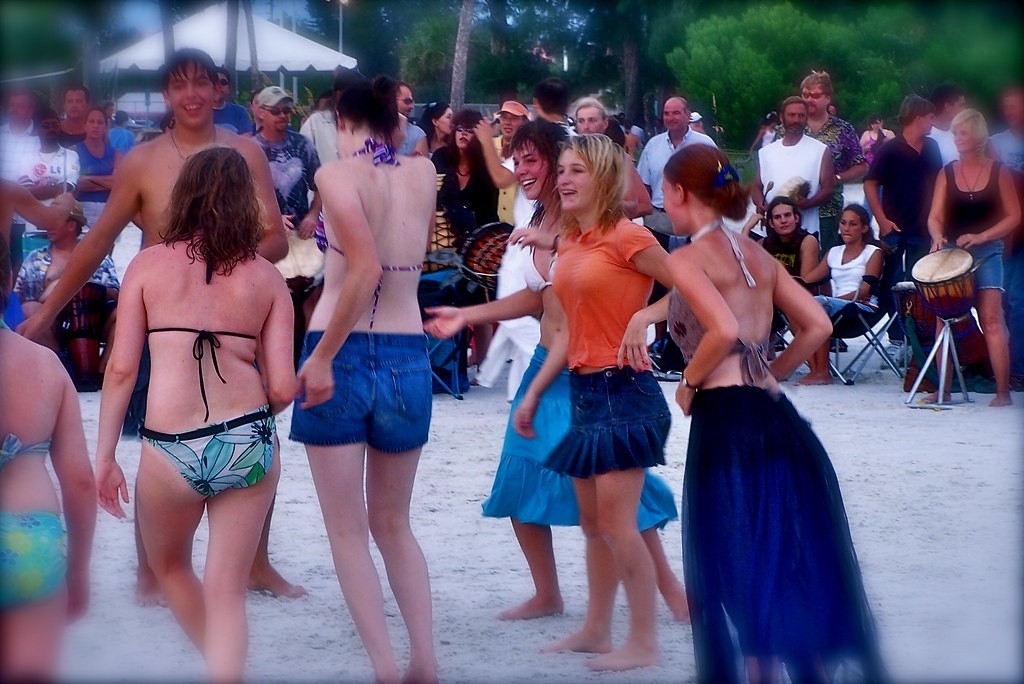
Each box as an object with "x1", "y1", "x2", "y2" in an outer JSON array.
[{"x1": 98, "y1": 0, "x2": 358, "y2": 99}]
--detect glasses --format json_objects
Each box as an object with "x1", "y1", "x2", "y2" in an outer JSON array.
[
  {"x1": 397, "y1": 97, "x2": 415, "y2": 105},
  {"x1": 803, "y1": 90, "x2": 825, "y2": 100},
  {"x1": 259, "y1": 107, "x2": 290, "y2": 115},
  {"x1": 41, "y1": 122, "x2": 59, "y2": 129},
  {"x1": 457, "y1": 125, "x2": 474, "y2": 133},
  {"x1": 220, "y1": 78, "x2": 228, "y2": 85},
  {"x1": 428, "y1": 102, "x2": 437, "y2": 117}
]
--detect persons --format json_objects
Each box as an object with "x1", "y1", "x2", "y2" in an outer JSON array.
[
  {"x1": 662, "y1": 143, "x2": 899, "y2": 684},
  {"x1": 0, "y1": 47, "x2": 678, "y2": 684},
  {"x1": 748, "y1": 69, "x2": 1024, "y2": 408}
]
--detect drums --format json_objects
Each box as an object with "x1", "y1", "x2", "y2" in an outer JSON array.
[
  {"x1": 55, "y1": 279, "x2": 111, "y2": 388},
  {"x1": 910, "y1": 248, "x2": 978, "y2": 324},
  {"x1": 456, "y1": 220, "x2": 515, "y2": 297}
]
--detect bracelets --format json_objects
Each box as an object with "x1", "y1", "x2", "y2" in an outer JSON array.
[
  {"x1": 835, "y1": 173, "x2": 842, "y2": 182},
  {"x1": 682, "y1": 369, "x2": 702, "y2": 389}
]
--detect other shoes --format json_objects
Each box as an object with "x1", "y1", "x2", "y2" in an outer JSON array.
[
  {"x1": 881, "y1": 339, "x2": 904, "y2": 369},
  {"x1": 775, "y1": 341, "x2": 784, "y2": 351},
  {"x1": 830, "y1": 339, "x2": 847, "y2": 352}
]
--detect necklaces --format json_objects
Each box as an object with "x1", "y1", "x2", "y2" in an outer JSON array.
[
  {"x1": 457, "y1": 170, "x2": 469, "y2": 176},
  {"x1": 38, "y1": 148, "x2": 59, "y2": 164},
  {"x1": 960, "y1": 158, "x2": 990, "y2": 200},
  {"x1": 169, "y1": 125, "x2": 217, "y2": 159}
]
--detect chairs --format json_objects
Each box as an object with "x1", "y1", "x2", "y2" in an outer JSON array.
[
  {"x1": 768, "y1": 275, "x2": 905, "y2": 385},
  {"x1": 416, "y1": 269, "x2": 478, "y2": 402}
]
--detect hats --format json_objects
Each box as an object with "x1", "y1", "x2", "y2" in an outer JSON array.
[
  {"x1": 70, "y1": 202, "x2": 87, "y2": 226},
  {"x1": 689, "y1": 112, "x2": 702, "y2": 123},
  {"x1": 258, "y1": 86, "x2": 293, "y2": 107},
  {"x1": 764, "y1": 113, "x2": 775, "y2": 124},
  {"x1": 497, "y1": 101, "x2": 529, "y2": 119},
  {"x1": 33, "y1": 108, "x2": 58, "y2": 124}
]
